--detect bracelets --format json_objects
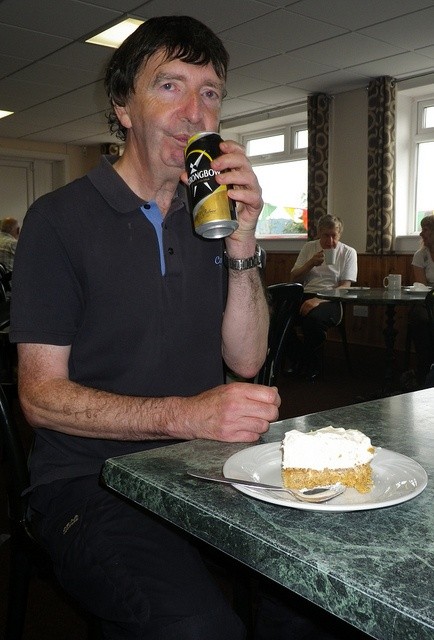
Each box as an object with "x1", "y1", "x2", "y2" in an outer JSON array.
[{"x1": 221, "y1": 243, "x2": 267, "y2": 271}]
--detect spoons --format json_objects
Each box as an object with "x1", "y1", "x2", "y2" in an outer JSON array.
[{"x1": 186, "y1": 469, "x2": 346, "y2": 503}]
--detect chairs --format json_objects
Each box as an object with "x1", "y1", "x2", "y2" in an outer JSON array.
[
  {"x1": 1, "y1": 263, "x2": 13, "y2": 329},
  {"x1": 1, "y1": 281, "x2": 304, "y2": 640}
]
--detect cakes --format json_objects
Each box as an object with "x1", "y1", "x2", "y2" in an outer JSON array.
[{"x1": 281, "y1": 425, "x2": 374, "y2": 494}]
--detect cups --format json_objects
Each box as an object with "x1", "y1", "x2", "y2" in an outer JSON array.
[
  {"x1": 383, "y1": 274, "x2": 401, "y2": 290},
  {"x1": 322, "y1": 248, "x2": 337, "y2": 265}
]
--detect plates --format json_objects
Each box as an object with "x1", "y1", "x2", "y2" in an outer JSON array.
[
  {"x1": 343, "y1": 287, "x2": 371, "y2": 292},
  {"x1": 403, "y1": 289, "x2": 431, "y2": 294},
  {"x1": 404, "y1": 286, "x2": 433, "y2": 289},
  {"x1": 223, "y1": 440, "x2": 428, "y2": 512}
]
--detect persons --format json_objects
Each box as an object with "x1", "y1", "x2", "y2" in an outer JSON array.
[
  {"x1": 283, "y1": 213, "x2": 358, "y2": 385},
  {"x1": 0, "y1": 218, "x2": 21, "y2": 273},
  {"x1": 410, "y1": 215, "x2": 434, "y2": 385},
  {"x1": 7, "y1": 14, "x2": 282, "y2": 640}
]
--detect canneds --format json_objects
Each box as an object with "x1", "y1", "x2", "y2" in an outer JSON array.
[{"x1": 182, "y1": 133, "x2": 239, "y2": 242}]
